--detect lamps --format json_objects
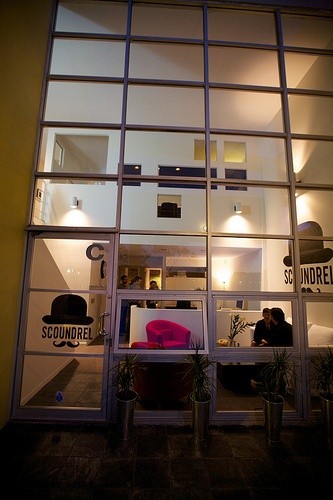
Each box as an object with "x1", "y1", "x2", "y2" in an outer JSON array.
[
  {"x1": 235, "y1": 202, "x2": 243, "y2": 213},
  {"x1": 70, "y1": 196, "x2": 78, "y2": 208}
]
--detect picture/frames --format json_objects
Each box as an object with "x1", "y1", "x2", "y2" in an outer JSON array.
[{"x1": 157, "y1": 194, "x2": 182, "y2": 219}]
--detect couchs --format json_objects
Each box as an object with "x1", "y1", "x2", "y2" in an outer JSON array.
[
  {"x1": 249, "y1": 319, "x2": 333, "y2": 397},
  {"x1": 146, "y1": 319, "x2": 191, "y2": 349},
  {"x1": 131, "y1": 342, "x2": 194, "y2": 407}
]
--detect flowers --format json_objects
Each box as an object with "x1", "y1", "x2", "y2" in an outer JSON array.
[{"x1": 228, "y1": 313, "x2": 256, "y2": 340}]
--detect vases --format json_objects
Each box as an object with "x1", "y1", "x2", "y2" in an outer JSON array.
[{"x1": 227, "y1": 339, "x2": 234, "y2": 347}]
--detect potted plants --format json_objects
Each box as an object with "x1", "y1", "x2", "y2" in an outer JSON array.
[
  {"x1": 312, "y1": 346, "x2": 333, "y2": 431},
  {"x1": 179, "y1": 340, "x2": 217, "y2": 456},
  {"x1": 106, "y1": 354, "x2": 140, "y2": 437},
  {"x1": 258, "y1": 347, "x2": 296, "y2": 442}
]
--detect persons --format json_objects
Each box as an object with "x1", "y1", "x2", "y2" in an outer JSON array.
[
  {"x1": 146, "y1": 281, "x2": 160, "y2": 309},
  {"x1": 119, "y1": 275, "x2": 129, "y2": 336},
  {"x1": 254, "y1": 307, "x2": 293, "y2": 348},
  {"x1": 127, "y1": 276, "x2": 142, "y2": 340}
]
